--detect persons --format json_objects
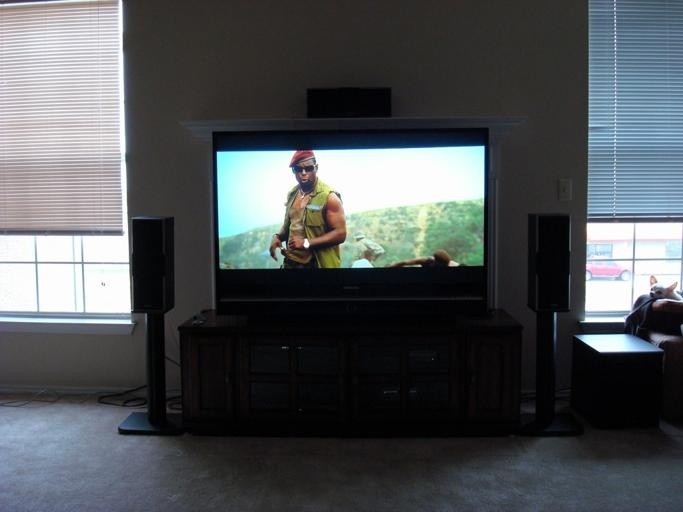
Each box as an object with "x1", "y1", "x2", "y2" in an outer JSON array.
[
  {"x1": 389, "y1": 249, "x2": 450, "y2": 267},
  {"x1": 352, "y1": 250, "x2": 374, "y2": 268},
  {"x1": 269, "y1": 150, "x2": 346, "y2": 268}
]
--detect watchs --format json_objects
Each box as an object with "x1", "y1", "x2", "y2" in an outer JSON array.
[{"x1": 303, "y1": 238, "x2": 310, "y2": 250}]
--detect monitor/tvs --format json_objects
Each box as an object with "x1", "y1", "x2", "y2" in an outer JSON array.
[{"x1": 212, "y1": 116, "x2": 498, "y2": 330}]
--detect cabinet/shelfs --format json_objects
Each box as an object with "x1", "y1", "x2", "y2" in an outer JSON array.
[
  {"x1": 569, "y1": 333, "x2": 666, "y2": 432},
  {"x1": 176, "y1": 308, "x2": 524, "y2": 440}
]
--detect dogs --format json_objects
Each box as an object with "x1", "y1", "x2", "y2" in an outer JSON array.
[{"x1": 648, "y1": 274, "x2": 682, "y2": 302}]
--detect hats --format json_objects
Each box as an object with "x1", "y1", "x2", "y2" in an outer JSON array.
[{"x1": 289, "y1": 150, "x2": 314, "y2": 166}]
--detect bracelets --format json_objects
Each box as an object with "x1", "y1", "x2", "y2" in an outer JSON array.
[{"x1": 273, "y1": 233, "x2": 281, "y2": 238}]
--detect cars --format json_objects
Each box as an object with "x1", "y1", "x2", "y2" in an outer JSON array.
[{"x1": 585, "y1": 257, "x2": 632, "y2": 282}]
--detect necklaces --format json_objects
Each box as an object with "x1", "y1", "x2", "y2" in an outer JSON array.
[{"x1": 301, "y1": 188, "x2": 313, "y2": 199}]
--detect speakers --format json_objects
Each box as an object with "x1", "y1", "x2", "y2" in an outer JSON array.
[
  {"x1": 306, "y1": 86, "x2": 391, "y2": 118},
  {"x1": 129, "y1": 217, "x2": 174, "y2": 314},
  {"x1": 526, "y1": 213, "x2": 570, "y2": 311}
]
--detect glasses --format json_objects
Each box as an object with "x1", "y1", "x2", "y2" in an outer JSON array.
[{"x1": 293, "y1": 164, "x2": 317, "y2": 173}]
